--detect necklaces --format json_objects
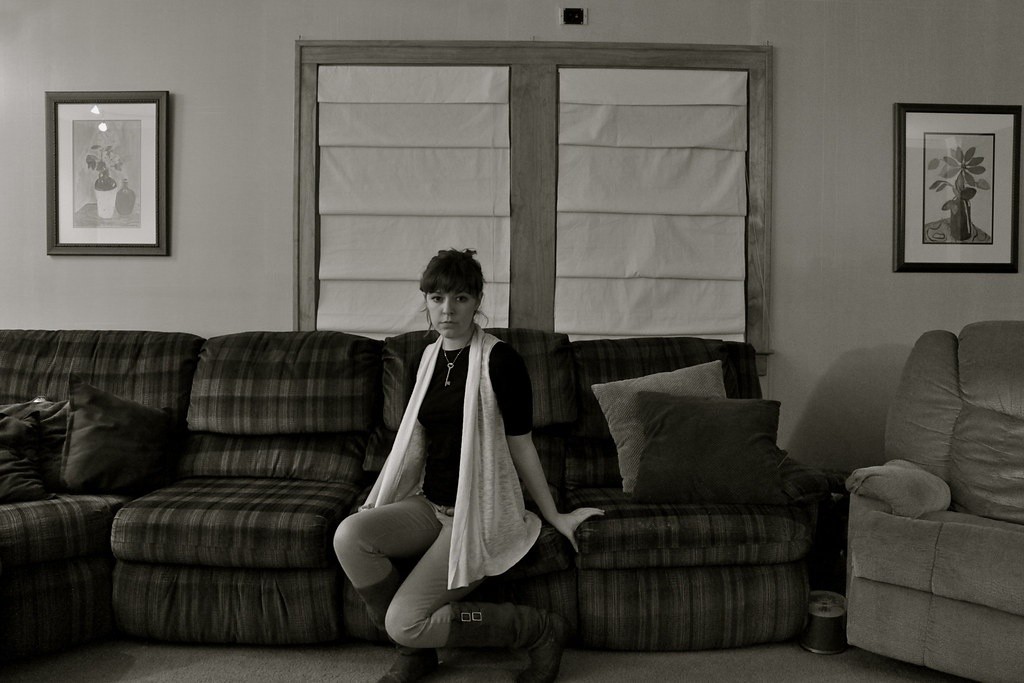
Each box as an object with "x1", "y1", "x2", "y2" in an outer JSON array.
[{"x1": 443, "y1": 332, "x2": 474, "y2": 386}]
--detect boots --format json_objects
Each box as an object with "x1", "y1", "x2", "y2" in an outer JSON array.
[
  {"x1": 440, "y1": 601, "x2": 566, "y2": 683},
  {"x1": 352, "y1": 565, "x2": 438, "y2": 683}
]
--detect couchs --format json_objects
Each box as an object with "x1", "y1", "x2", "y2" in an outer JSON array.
[
  {"x1": 0, "y1": 328, "x2": 821, "y2": 683},
  {"x1": 842, "y1": 319, "x2": 1024, "y2": 683}
]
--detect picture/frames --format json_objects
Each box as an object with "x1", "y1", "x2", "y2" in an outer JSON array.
[
  {"x1": 44, "y1": 90, "x2": 171, "y2": 258},
  {"x1": 892, "y1": 102, "x2": 1022, "y2": 274}
]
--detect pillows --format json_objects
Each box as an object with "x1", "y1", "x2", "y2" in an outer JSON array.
[
  {"x1": 634, "y1": 390, "x2": 782, "y2": 506},
  {"x1": 58, "y1": 380, "x2": 194, "y2": 498},
  {"x1": 591, "y1": 360, "x2": 726, "y2": 494},
  {"x1": 0, "y1": 395, "x2": 69, "y2": 500}
]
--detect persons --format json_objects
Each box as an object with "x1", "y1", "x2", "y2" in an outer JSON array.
[{"x1": 333, "y1": 247, "x2": 606, "y2": 683}]
